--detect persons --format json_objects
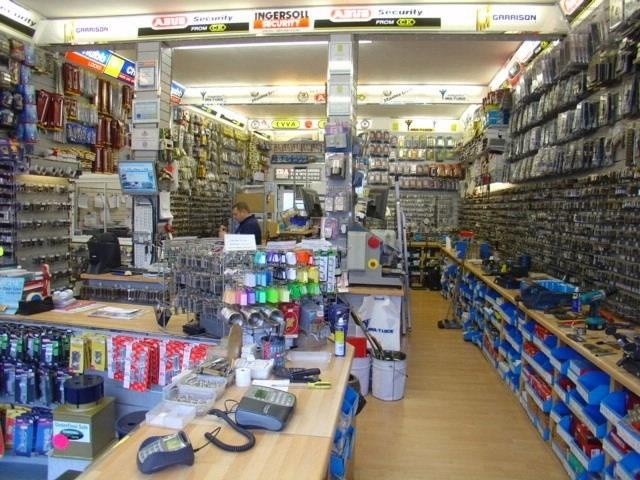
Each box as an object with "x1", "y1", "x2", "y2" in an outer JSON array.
[{"x1": 216, "y1": 201, "x2": 262, "y2": 244}]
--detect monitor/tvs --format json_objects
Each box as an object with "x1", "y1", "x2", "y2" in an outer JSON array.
[{"x1": 117, "y1": 159, "x2": 156, "y2": 195}]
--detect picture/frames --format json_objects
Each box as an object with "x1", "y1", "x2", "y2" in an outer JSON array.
[
  {"x1": 134, "y1": 60, "x2": 159, "y2": 92},
  {"x1": 131, "y1": 97, "x2": 161, "y2": 124}
]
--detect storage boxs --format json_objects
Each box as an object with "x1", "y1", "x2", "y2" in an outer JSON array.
[{"x1": 52, "y1": 397, "x2": 117, "y2": 458}]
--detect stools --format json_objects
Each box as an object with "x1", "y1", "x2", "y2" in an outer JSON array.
[{"x1": 43, "y1": 437, "x2": 120, "y2": 480}]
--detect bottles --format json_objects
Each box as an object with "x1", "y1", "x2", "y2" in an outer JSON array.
[
  {"x1": 333, "y1": 317, "x2": 347, "y2": 357},
  {"x1": 572, "y1": 286, "x2": 583, "y2": 313}
]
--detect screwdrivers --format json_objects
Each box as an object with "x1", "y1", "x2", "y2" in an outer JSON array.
[{"x1": 271, "y1": 382, "x2": 332, "y2": 389}]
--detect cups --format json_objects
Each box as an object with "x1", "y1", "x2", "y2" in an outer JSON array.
[
  {"x1": 259, "y1": 305, "x2": 283, "y2": 326},
  {"x1": 220, "y1": 306, "x2": 243, "y2": 328},
  {"x1": 239, "y1": 307, "x2": 263, "y2": 328},
  {"x1": 261, "y1": 334, "x2": 285, "y2": 373},
  {"x1": 234, "y1": 368, "x2": 252, "y2": 387}
]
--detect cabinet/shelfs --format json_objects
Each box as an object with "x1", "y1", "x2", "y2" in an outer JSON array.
[{"x1": 398, "y1": 241, "x2": 446, "y2": 290}]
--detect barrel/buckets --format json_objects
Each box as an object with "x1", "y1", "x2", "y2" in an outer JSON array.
[
  {"x1": 357, "y1": 294, "x2": 399, "y2": 350},
  {"x1": 350, "y1": 353, "x2": 371, "y2": 396},
  {"x1": 372, "y1": 351, "x2": 408, "y2": 401}
]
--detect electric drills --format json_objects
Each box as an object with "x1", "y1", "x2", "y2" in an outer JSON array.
[{"x1": 580, "y1": 284, "x2": 624, "y2": 330}]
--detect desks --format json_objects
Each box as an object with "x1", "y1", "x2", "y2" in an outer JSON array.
[
  {"x1": 0, "y1": 297, "x2": 356, "y2": 480},
  {"x1": 338, "y1": 285, "x2": 405, "y2": 353},
  {"x1": 80, "y1": 268, "x2": 169, "y2": 303}
]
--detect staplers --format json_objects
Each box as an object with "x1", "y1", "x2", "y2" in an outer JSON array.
[{"x1": 288, "y1": 368, "x2": 321, "y2": 382}]
